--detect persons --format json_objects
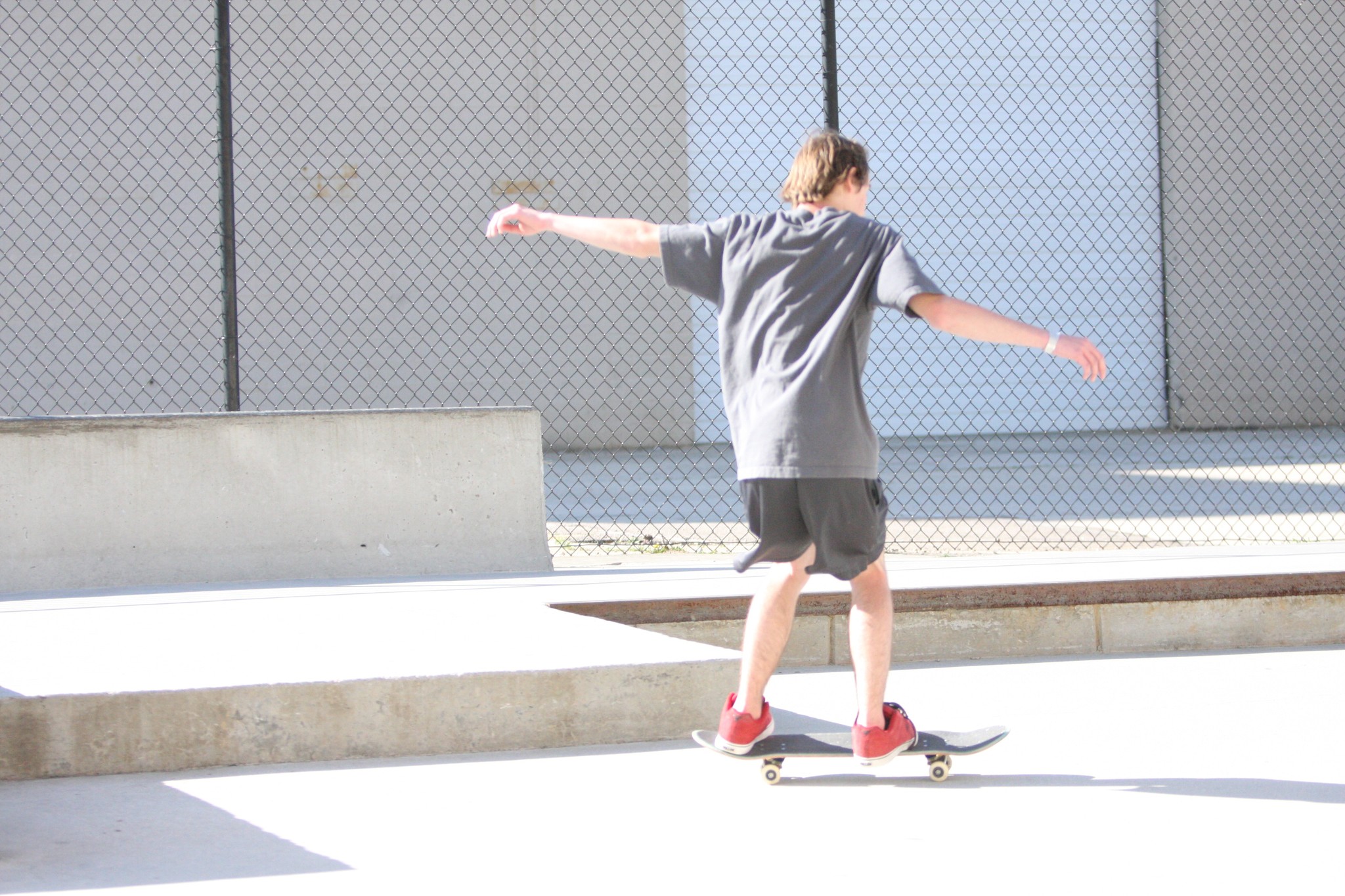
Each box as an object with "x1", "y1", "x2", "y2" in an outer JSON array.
[{"x1": 483, "y1": 135, "x2": 1107, "y2": 769}]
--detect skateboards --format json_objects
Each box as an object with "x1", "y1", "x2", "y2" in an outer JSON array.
[{"x1": 691, "y1": 729, "x2": 1013, "y2": 786}]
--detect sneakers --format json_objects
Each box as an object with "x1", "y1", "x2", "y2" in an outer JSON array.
[
  {"x1": 853, "y1": 702, "x2": 917, "y2": 764},
  {"x1": 714, "y1": 693, "x2": 774, "y2": 754}
]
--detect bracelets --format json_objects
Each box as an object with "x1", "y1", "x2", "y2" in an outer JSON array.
[{"x1": 1044, "y1": 331, "x2": 1060, "y2": 354}]
